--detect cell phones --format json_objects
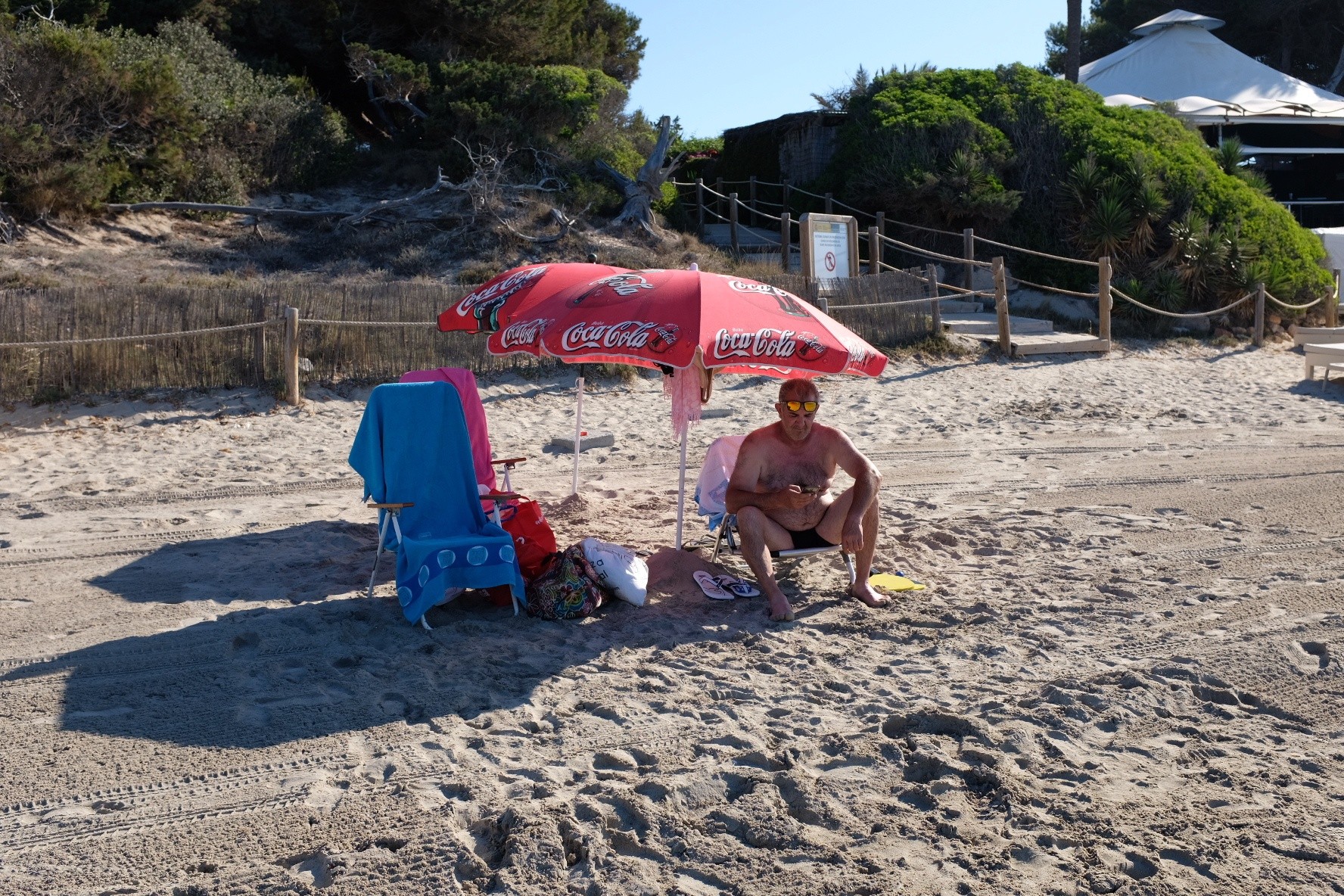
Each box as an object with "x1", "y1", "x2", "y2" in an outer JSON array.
[{"x1": 801, "y1": 485, "x2": 820, "y2": 497}]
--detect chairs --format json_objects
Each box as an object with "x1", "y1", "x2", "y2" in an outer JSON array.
[
  {"x1": 398, "y1": 368, "x2": 528, "y2": 579},
  {"x1": 694, "y1": 433, "x2": 860, "y2": 592},
  {"x1": 349, "y1": 382, "x2": 525, "y2": 629}
]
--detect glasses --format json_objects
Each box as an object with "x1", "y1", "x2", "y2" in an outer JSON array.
[{"x1": 780, "y1": 400, "x2": 819, "y2": 412}]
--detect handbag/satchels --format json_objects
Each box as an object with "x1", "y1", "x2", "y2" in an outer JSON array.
[
  {"x1": 484, "y1": 489, "x2": 556, "y2": 606},
  {"x1": 526, "y1": 545, "x2": 610, "y2": 620},
  {"x1": 580, "y1": 537, "x2": 649, "y2": 607}
]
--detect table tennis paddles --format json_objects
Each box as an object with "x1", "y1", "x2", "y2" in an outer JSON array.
[
  {"x1": 895, "y1": 570, "x2": 926, "y2": 591},
  {"x1": 869, "y1": 567, "x2": 915, "y2": 591}
]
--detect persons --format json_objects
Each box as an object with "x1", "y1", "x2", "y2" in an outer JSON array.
[{"x1": 724, "y1": 378, "x2": 891, "y2": 622}]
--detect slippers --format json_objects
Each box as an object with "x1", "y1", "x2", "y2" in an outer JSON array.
[
  {"x1": 694, "y1": 571, "x2": 735, "y2": 600},
  {"x1": 717, "y1": 575, "x2": 760, "y2": 596}
]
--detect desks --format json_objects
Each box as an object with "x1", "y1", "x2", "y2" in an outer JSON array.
[{"x1": 1303, "y1": 342, "x2": 1344, "y2": 388}]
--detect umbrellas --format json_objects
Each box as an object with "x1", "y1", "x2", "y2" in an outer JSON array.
[
  {"x1": 486, "y1": 263, "x2": 889, "y2": 551},
  {"x1": 436, "y1": 251, "x2": 635, "y2": 492}
]
